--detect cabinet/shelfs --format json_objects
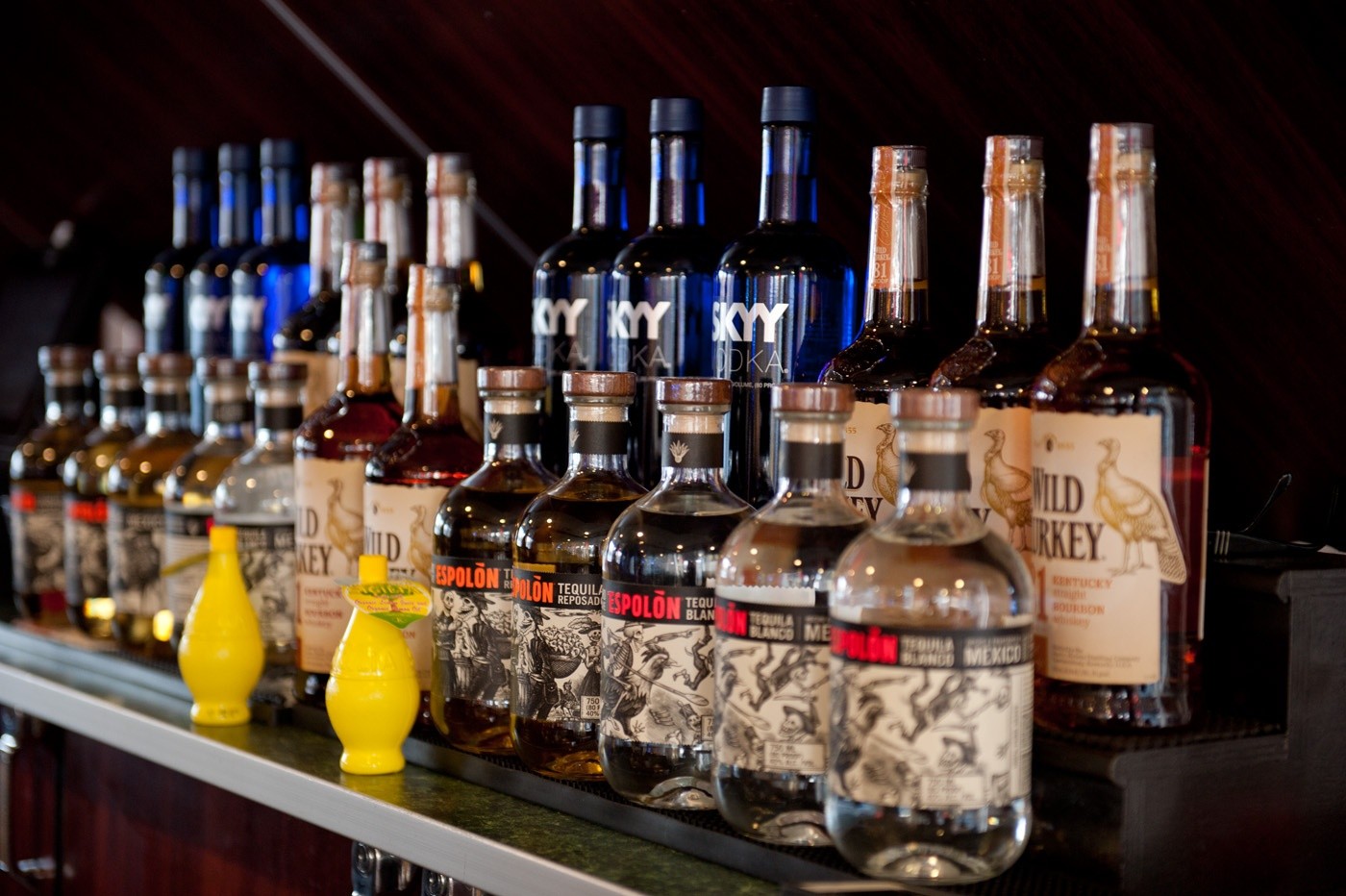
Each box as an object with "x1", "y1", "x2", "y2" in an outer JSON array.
[{"x1": 1035, "y1": 566, "x2": 1346, "y2": 896}]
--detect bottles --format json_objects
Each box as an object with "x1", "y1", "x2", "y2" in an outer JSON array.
[
  {"x1": 531, "y1": 102, "x2": 642, "y2": 476},
  {"x1": 62, "y1": 348, "x2": 144, "y2": 639},
  {"x1": 216, "y1": 363, "x2": 309, "y2": 708},
  {"x1": 10, "y1": 346, "x2": 93, "y2": 635},
  {"x1": 712, "y1": 383, "x2": 875, "y2": 845},
  {"x1": 271, "y1": 160, "x2": 360, "y2": 420},
  {"x1": 714, "y1": 85, "x2": 857, "y2": 508},
  {"x1": 431, "y1": 367, "x2": 559, "y2": 752},
  {"x1": 511, "y1": 371, "x2": 650, "y2": 779},
  {"x1": 612, "y1": 98, "x2": 735, "y2": 493},
  {"x1": 143, "y1": 136, "x2": 312, "y2": 361},
  {"x1": 108, "y1": 351, "x2": 201, "y2": 662},
  {"x1": 162, "y1": 358, "x2": 254, "y2": 678},
  {"x1": 311, "y1": 158, "x2": 412, "y2": 416},
  {"x1": 1033, "y1": 121, "x2": 1211, "y2": 746},
  {"x1": 0, "y1": 703, "x2": 57, "y2": 877},
  {"x1": 178, "y1": 526, "x2": 265, "y2": 727},
  {"x1": 601, "y1": 377, "x2": 755, "y2": 811},
  {"x1": 325, "y1": 555, "x2": 420, "y2": 775},
  {"x1": 929, "y1": 135, "x2": 1066, "y2": 724},
  {"x1": 364, "y1": 266, "x2": 482, "y2": 742},
  {"x1": 390, "y1": 154, "x2": 505, "y2": 438},
  {"x1": 817, "y1": 144, "x2": 958, "y2": 528},
  {"x1": 825, "y1": 387, "x2": 1035, "y2": 884},
  {"x1": 295, "y1": 240, "x2": 404, "y2": 714}
]
